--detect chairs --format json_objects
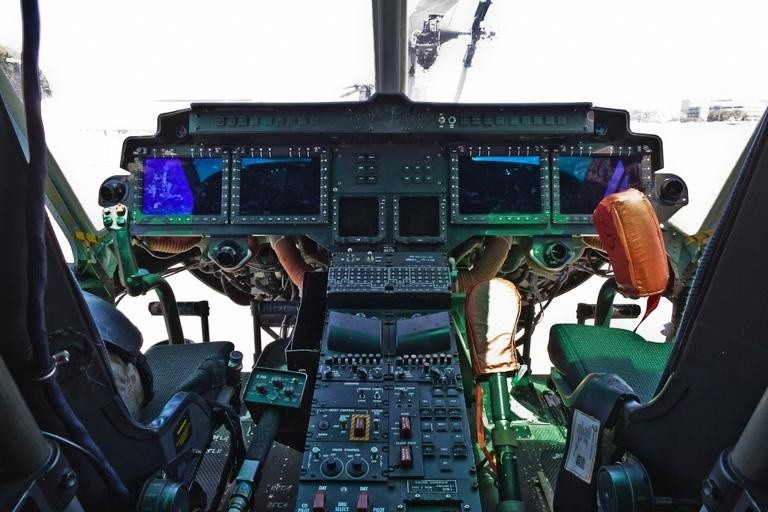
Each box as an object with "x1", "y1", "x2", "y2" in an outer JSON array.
[
  {"x1": 537, "y1": 107, "x2": 767, "y2": 512},
  {"x1": 0, "y1": 71, "x2": 246, "y2": 512}
]
